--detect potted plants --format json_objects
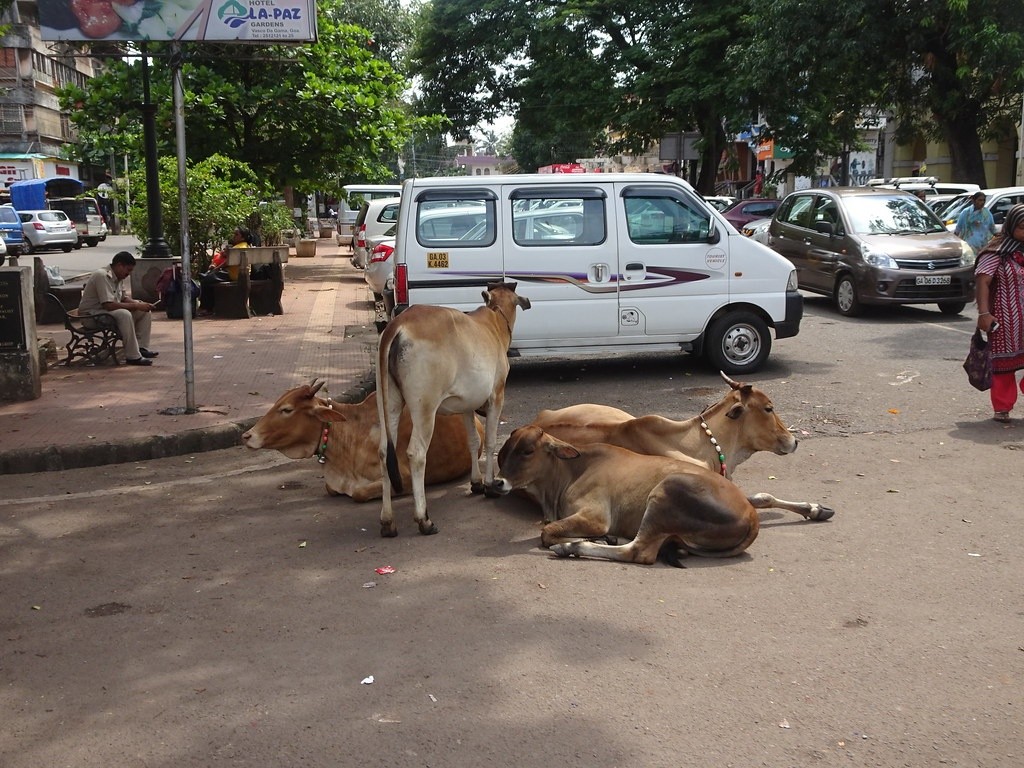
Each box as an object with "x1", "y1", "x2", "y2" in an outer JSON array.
[{"x1": 224, "y1": 200, "x2": 298, "y2": 267}]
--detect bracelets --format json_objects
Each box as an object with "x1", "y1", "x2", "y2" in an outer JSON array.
[{"x1": 978, "y1": 312, "x2": 992, "y2": 318}]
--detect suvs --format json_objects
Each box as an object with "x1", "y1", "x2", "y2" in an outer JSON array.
[
  {"x1": 0, "y1": 206, "x2": 25, "y2": 258},
  {"x1": 349, "y1": 198, "x2": 479, "y2": 269}
]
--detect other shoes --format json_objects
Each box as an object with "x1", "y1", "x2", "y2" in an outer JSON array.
[{"x1": 993, "y1": 411, "x2": 1011, "y2": 423}]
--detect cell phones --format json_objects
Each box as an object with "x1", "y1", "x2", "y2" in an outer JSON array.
[
  {"x1": 991, "y1": 322, "x2": 999, "y2": 333},
  {"x1": 153, "y1": 300, "x2": 162, "y2": 306}
]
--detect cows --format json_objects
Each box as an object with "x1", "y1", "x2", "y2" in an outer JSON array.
[{"x1": 240, "y1": 280, "x2": 836, "y2": 571}]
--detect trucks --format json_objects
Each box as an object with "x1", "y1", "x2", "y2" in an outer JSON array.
[{"x1": 3, "y1": 177, "x2": 88, "y2": 250}]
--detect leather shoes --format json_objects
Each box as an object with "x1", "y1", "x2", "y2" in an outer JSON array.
[
  {"x1": 125, "y1": 357, "x2": 153, "y2": 366},
  {"x1": 139, "y1": 347, "x2": 160, "y2": 358}
]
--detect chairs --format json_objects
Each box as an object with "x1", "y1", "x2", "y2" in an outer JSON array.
[{"x1": 823, "y1": 207, "x2": 838, "y2": 224}]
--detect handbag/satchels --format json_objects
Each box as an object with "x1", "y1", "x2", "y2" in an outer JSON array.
[{"x1": 963, "y1": 326, "x2": 993, "y2": 392}]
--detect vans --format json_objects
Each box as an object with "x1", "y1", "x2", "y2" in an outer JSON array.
[
  {"x1": 335, "y1": 183, "x2": 402, "y2": 246},
  {"x1": 381, "y1": 170, "x2": 804, "y2": 376}
]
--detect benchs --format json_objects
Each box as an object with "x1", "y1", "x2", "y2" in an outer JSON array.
[
  {"x1": 317, "y1": 218, "x2": 335, "y2": 238},
  {"x1": 293, "y1": 228, "x2": 317, "y2": 257},
  {"x1": 46, "y1": 291, "x2": 124, "y2": 367},
  {"x1": 333, "y1": 224, "x2": 354, "y2": 247}
]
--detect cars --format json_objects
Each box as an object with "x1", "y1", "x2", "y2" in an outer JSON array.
[
  {"x1": 363, "y1": 208, "x2": 571, "y2": 304},
  {"x1": 767, "y1": 186, "x2": 979, "y2": 317},
  {"x1": 382, "y1": 205, "x2": 583, "y2": 321},
  {"x1": 703, "y1": 195, "x2": 829, "y2": 247},
  {"x1": 859, "y1": 176, "x2": 1024, "y2": 235},
  {"x1": 83, "y1": 197, "x2": 109, "y2": 247},
  {"x1": 15, "y1": 210, "x2": 79, "y2": 254}
]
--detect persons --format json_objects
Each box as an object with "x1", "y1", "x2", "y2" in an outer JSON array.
[
  {"x1": 553, "y1": 166, "x2": 564, "y2": 174},
  {"x1": 954, "y1": 192, "x2": 998, "y2": 257},
  {"x1": 78, "y1": 251, "x2": 158, "y2": 366},
  {"x1": 196, "y1": 226, "x2": 251, "y2": 316},
  {"x1": 973, "y1": 202, "x2": 1024, "y2": 422},
  {"x1": 754, "y1": 170, "x2": 764, "y2": 195},
  {"x1": 328, "y1": 206, "x2": 334, "y2": 218}
]
